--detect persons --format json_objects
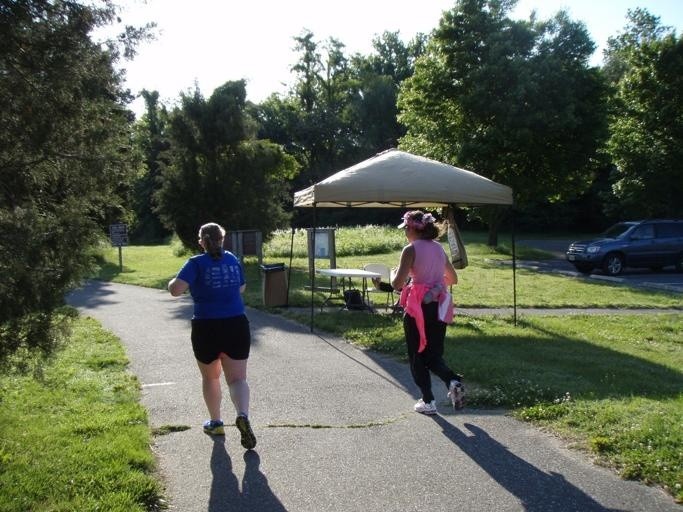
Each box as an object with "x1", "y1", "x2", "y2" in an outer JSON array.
[
  {"x1": 168, "y1": 222, "x2": 257, "y2": 449},
  {"x1": 389, "y1": 210, "x2": 466, "y2": 414}
]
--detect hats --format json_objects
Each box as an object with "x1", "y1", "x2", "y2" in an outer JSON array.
[{"x1": 397, "y1": 212, "x2": 423, "y2": 230}]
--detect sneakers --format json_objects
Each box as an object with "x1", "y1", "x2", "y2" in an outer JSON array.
[
  {"x1": 414, "y1": 398, "x2": 437, "y2": 416},
  {"x1": 235, "y1": 414, "x2": 257, "y2": 449},
  {"x1": 448, "y1": 380, "x2": 465, "y2": 411},
  {"x1": 204, "y1": 420, "x2": 225, "y2": 436}
]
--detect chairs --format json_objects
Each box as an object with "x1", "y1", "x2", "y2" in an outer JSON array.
[
  {"x1": 364, "y1": 263, "x2": 396, "y2": 315},
  {"x1": 391, "y1": 267, "x2": 403, "y2": 296}
]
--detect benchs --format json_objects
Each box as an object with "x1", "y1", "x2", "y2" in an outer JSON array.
[{"x1": 305, "y1": 285, "x2": 340, "y2": 315}]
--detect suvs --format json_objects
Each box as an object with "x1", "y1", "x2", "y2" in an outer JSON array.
[{"x1": 565, "y1": 218, "x2": 682, "y2": 275}]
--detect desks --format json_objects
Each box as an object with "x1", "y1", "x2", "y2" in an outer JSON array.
[{"x1": 316, "y1": 268, "x2": 382, "y2": 315}]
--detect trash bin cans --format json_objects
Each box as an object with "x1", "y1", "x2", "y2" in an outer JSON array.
[{"x1": 259, "y1": 262, "x2": 288, "y2": 306}]
